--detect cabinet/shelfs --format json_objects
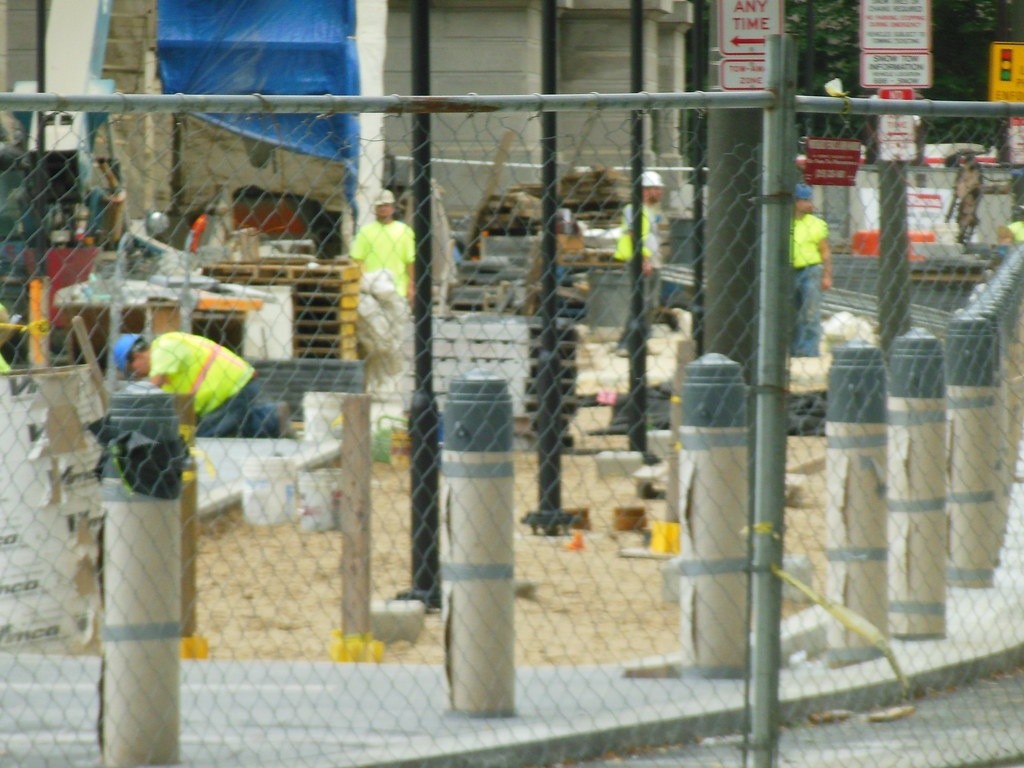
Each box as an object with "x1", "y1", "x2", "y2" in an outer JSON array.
[{"x1": 205, "y1": 261, "x2": 363, "y2": 363}]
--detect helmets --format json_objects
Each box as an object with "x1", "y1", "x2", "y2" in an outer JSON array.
[
  {"x1": 374, "y1": 189, "x2": 397, "y2": 205},
  {"x1": 110, "y1": 334, "x2": 143, "y2": 374},
  {"x1": 640, "y1": 170, "x2": 665, "y2": 188},
  {"x1": 794, "y1": 183, "x2": 815, "y2": 199}
]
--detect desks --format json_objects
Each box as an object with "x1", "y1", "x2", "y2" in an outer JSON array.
[{"x1": 63, "y1": 297, "x2": 197, "y2": 372}]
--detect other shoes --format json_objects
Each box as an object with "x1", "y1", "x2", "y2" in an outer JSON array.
[{"x1": 275, "y1": 399, "x2": 291, "y2": 438}]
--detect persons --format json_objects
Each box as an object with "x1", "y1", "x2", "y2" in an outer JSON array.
[
  {"x1": 112, "y1": 331, "x2": 262, "y2": 438},
  {"x1": 788, "y1": 184, "x2": 831, "y2": 357},
  {"x1": 613, "y1": 171, "x2": 665, "y2": 357},
  {"x1": 348, "y1": 189, "x2": 415, "y2": 391}
]
